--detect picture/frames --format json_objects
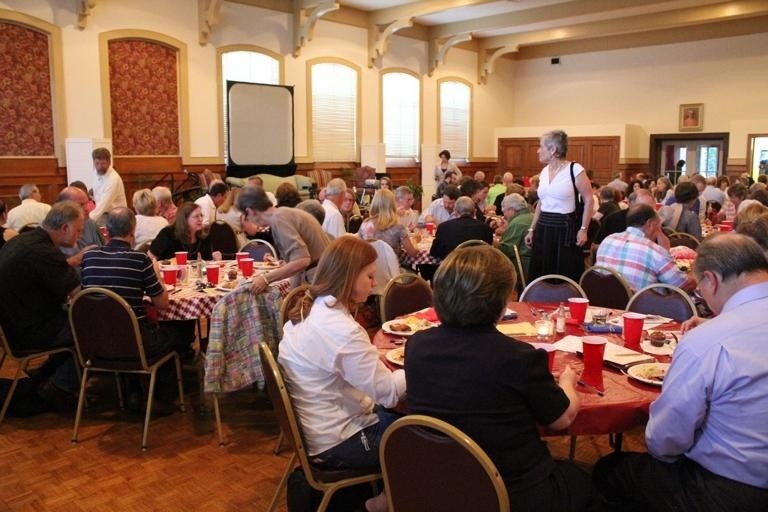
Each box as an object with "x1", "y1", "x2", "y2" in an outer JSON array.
[{"x1": 678, "y1": 103, "x2": 704, "y2": 132}]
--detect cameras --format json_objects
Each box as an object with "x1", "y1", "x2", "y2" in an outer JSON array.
[{"x1": 301, "y1": 181, "x2": 320, "y2": 199}]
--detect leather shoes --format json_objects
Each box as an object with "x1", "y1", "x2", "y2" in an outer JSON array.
[
  {"x1": 142, "y1": 398, "x2": 177, "y2": 414},
  {"x1": 127, "y1": 391, "x2": 144, "y2": 414}
]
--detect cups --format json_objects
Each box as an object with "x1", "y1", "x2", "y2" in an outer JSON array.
[
  {"x1": 534, "y1": 298, "x2": 645, "y2": 372},
  {"x1": 164, "y1": 252, "x2": 254, "y2": 287},
  {"x1": 573, "y1": 370, "x2": 606, "y2": 394},
  {"x1": 424, "y1": 222, "x2": 434, "y2": 235},
  {"x1": 720, "y1": 225, "x2": 730, "y2": 233}
]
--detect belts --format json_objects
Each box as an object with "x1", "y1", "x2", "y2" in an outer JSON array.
[{"x1": 305, "y1": 259, "x2": 319, "y2": 271}]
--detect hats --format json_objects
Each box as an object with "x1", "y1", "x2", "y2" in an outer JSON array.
[{"x1": 657, "y1": 202, "x2": 683, "y2": 230}]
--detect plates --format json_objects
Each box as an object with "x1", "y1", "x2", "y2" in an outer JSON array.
[
  {"x1": 161, "y1": 284, "x2": 174, "y2": 291},
  {"x1": 382, "y1": 319, "x2": 438, "y2": 366},
  {"x1": 627, "y1": 338, "x2": 674, "y2": 386},
  {"x1": 158, "y1": 260, "x2": 173, "y2": 268},
  {"x1": 253, "y1": 262, "x2": 280, "y2": 269},
  {"x1": 216, "y1": 282, "x2": 236, "y2": 291}
]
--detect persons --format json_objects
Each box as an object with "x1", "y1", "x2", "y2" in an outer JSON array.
[
  {"x1": 274, "y1": 237, "x2": 413, "y2": 511},
  {"x1": 589, "y1": 231, "x2": 768, "y2": 509},
  {"x1": 0, "y1": 126, "x2": 768, "y2": 422},
  {"x1": 402, "y1": 246, "x2": 588, "y2": 512}
]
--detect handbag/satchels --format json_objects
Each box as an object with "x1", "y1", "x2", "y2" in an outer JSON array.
[{"x1": 564, "y1": 204, "x2": 593, "y2": 247}]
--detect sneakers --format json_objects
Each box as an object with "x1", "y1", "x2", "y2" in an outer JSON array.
[
  {"x1": 35, "y1": 378, "x2": 57, "y2": 400},
  {"x1": 63, "y1": 389, "x2": 71, "y2": 400}
]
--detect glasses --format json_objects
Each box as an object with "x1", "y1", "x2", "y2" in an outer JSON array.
[
  {"x1": 191, "y1": 215, "x2": 205, "y2": 222},
  {"x1": 244, "y1": 213, "x2": 248, "y2": 221},
  {"x1": 404, "y1": 199, "x2": 415, "y2": 203},
  {"x1": 693, "y1": 276, "x2": 704, "y2": 300}
]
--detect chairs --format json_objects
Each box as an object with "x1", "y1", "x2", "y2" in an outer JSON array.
[
  {"x1": 519, "y1": 274, "x2": 589, "y2": 303},
  {"x1": 199, "y1": 350, "x2": 225, "y2": 449},
  {"x1": 236, "y1": 239, "x2": 278, "y2": 261},
  {"x1": 380, "y1": 274, "x2": 434, "y2": 326},
  {"x1": 258, "y1": 341, "x2": 382, "y2": 512},
  {"x1": 279, "y1": 285, "x2": 313, "y2": 338},
  {"x1": 0, "y1": 326, "x2": 89, "y2": 424},
  {"x1": 625, "y1": 283, "x2": 697, "y2": 320},
  {"x1": 378, "y1": 415, "x2": 511, "y2": 511},
  {"x1": 667, "y1": 232, "x2": 701, "y2": 251},
  {"x1": 68, "y1": 288, "x2": 186, "y2": 452},
  {"x1": 579, "y1": 265, "x2": 632, "y2": 311},
  {"x1": 454, "y1": 239, "x2": 488, "y2": 249}
]
